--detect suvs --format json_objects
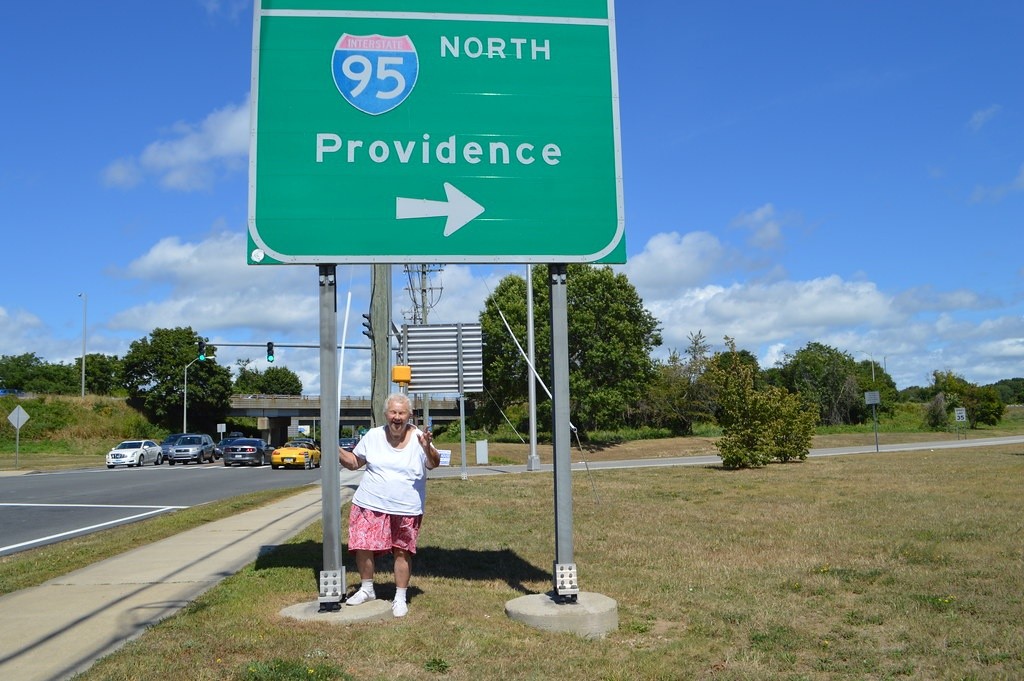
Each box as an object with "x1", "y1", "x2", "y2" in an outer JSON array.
[
  {"x1": 168, "y1": 434, "x2": 216, "y2": 466},
  {"x1": 160, "y1": 434, "x2": 182, "y2": 455}
]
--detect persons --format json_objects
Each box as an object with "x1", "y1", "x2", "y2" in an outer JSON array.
[{"x1": 339, "y1": 393, "x2": 441, "y2": 618}]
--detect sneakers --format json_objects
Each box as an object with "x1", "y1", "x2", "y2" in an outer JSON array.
[
  {"x1": 346, "y1": 587, "x2": 376, "y2": 606},
  {"x1": 392, "y1": 597, "x2": 408, "y2": 616}
]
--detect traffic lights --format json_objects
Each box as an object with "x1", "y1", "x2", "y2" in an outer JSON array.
[
  {"x1": 197, "y1": 340, "x2": 207, "y2": 362},
  {"x1": 267, "y1": 343, "x2": 274, "y2": 362}
]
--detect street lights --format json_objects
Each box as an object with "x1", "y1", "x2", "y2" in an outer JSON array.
[
  {"x1": 77, "y1": 291, "x2": 87, "y2": 396},
  {"x1": 183, "y1": 355, "x2": 216, "y2": 433},
  {"x1": 855, "y1": 349, "x2": 879, "y2": 453}
]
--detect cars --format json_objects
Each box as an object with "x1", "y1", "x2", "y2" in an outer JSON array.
[
  {"x1": 340, "y1": 438, "x2": 359, "y2": 452},
  {"x1": 216, "y1": 431, "x2": 246, "y2": 460},
  {"x1": 223, "y1": 438, "x2": 274, "y2": 466},
  {"x1": 106, "y1": 440, "x2": 163, "y2": 469},
  {"x1": 271, "y1": 442, "x2": 321, "y2": 469}
]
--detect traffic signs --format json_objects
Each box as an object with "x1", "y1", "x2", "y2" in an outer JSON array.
[{"x1": 250, "y1": 0, "x2": 623, "y2": 260}]
